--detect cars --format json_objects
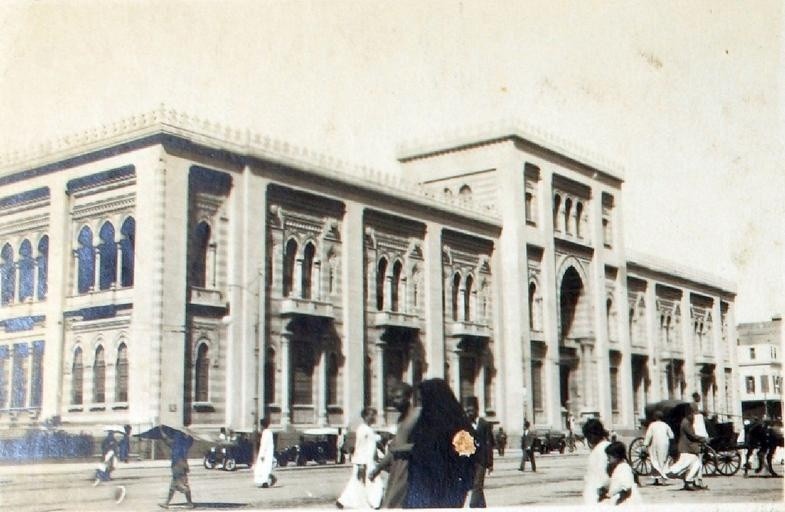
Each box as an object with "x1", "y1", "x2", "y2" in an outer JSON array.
[
  {"x1": 202, "y1": 429, "x2": 262, "y2": 472},
  {"x1": 277, "y1": 427, "x2": 348, "y2": 468}
]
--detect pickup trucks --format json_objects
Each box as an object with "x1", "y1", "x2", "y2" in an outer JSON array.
[{"x1": 530, "y1": 427, "x2": 566, "y2": 457}]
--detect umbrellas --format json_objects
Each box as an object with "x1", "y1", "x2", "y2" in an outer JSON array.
[{"x1": 104, "y1": 424, "x2": 126, "y2": 436}]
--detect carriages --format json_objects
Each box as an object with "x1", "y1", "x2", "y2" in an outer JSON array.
[{"x1": 627, "y1": 398, "x2": 783, "y2": 481}]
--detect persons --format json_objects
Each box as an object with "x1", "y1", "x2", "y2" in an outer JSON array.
[
  {"x1": 334, "y1": 377, "x2": 508, "y2": 508},
  {"x1": 518, "y1": 420, "x2": 537, "y2": 473},
  {"x1": 218, "y1": 427, "x2": 226, "y2": 454},
  {"x1": 253, "y1": 418, "x2": 280, "y2": 488},
  {"x1": 643, "y1": 391, "x2": 710, "y2": 491},
  {"x1": 596, "y1": 441, "x2": 642, "y2": 504},
  {"x1": 153, "y1": 424, "x2": 195, "y2": 510},
  {"x1": 91, "y1": 431, "x2": 130, "y2": 486},
  {"x1": 581, "y1": 418, "x2": 613, "y2": 504}
]
